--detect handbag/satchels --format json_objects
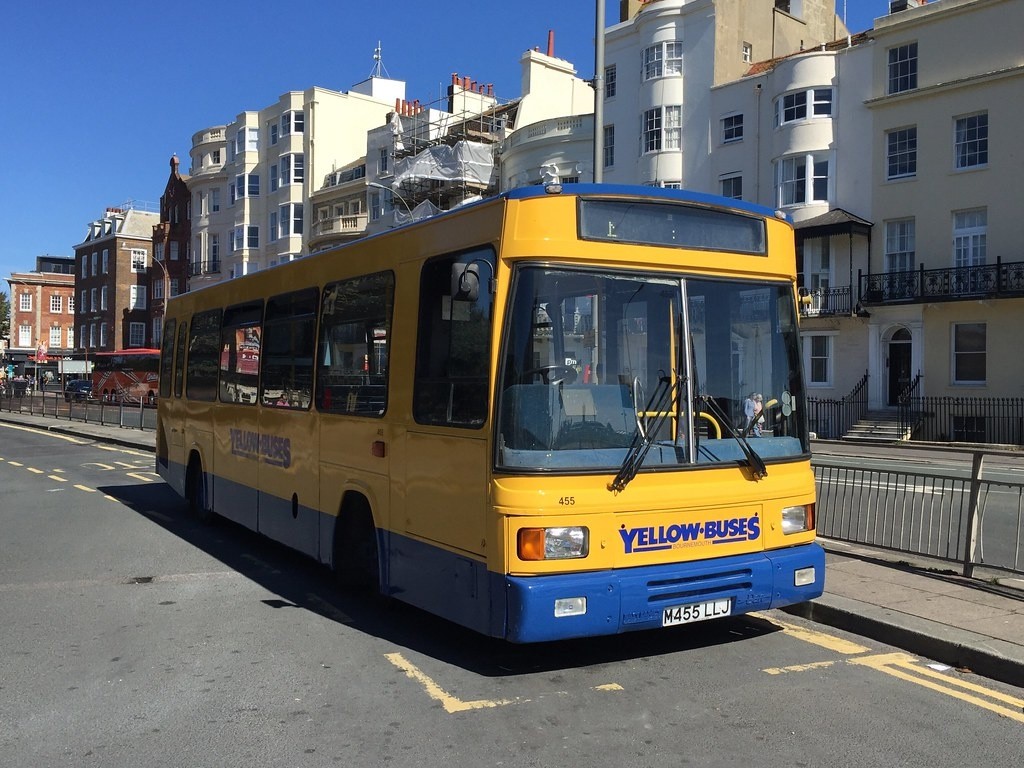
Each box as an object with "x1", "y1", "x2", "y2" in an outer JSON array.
[{"x1": 758, "y1": 415, "x2": 765, "y2": 423}]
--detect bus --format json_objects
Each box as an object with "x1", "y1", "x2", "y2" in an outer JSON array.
[
  {"x1": 155, "y1": 182, "x2": 825, "y2": 646},
  {"x1": 90, "y1": 349, "x2": 159, "y2": 408}
]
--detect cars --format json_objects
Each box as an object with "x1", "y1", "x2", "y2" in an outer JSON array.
[{"x1": 65, "y1": 379, "x2": 91, "y2": 401}]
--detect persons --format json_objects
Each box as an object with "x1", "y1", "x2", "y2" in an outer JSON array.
[
  {"x1": 744, "y1": 390, "x2": 764, "y2": 438},
  {"x1": 9, "y1": 373, "x2": 48, "y2": 392}
]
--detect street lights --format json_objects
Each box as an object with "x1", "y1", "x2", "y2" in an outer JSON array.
[
  {"x1": 3, "y1": 277, "x2": 38, "y2": 394},
  {"x1": 119, "y1": 248, "x2": 168, "y2": 313}
]
